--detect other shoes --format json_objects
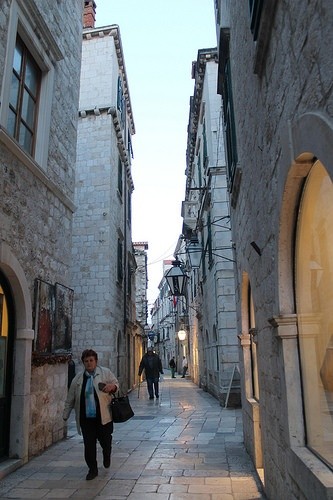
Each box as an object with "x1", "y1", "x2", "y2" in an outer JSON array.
[
  {"x1": 155, "y1": 394, "x2": 159, "y2": 398},
  {"x1": 103, "y1": 451, "x2": 110, "y2": 467},
  {"x1": 150, "y1": 394, "x2": 153, "y2": 399},
  {"x1": 86, "y1": 467, "x2": 98, "y2": 480}
]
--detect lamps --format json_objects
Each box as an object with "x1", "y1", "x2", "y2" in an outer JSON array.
[
  {"x1": 167, "y1": 291, "x2": 198, "y2": 313},
  {"x1": 183, "y1": 215, "x2": 232, "y2": 269},
  {"x1": 163, "y1": 246, "x2": 238, "y2": 296}
]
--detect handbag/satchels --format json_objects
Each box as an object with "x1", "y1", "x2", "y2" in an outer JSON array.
[{"x1": 110, "y1": 390, "x2": 134, "y2": 423}]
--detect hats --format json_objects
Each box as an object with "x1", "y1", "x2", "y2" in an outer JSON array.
[{"x1": 147, "y1": 347, "x2": 153, "y2": 351}]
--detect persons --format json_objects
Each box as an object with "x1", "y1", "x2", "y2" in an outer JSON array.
[
  {"x1": 181, "y1": 356, "x2": 188, "y2": 378},
  {"x1": 62, "y1": 349, "x2": 120, "y2": 480},
  {"x1": 169, "y1": 357, "x2": 176, "y2": 378},
  {"x1": 138, "y1": 347, "x2": 164, "y2": 399}
]
